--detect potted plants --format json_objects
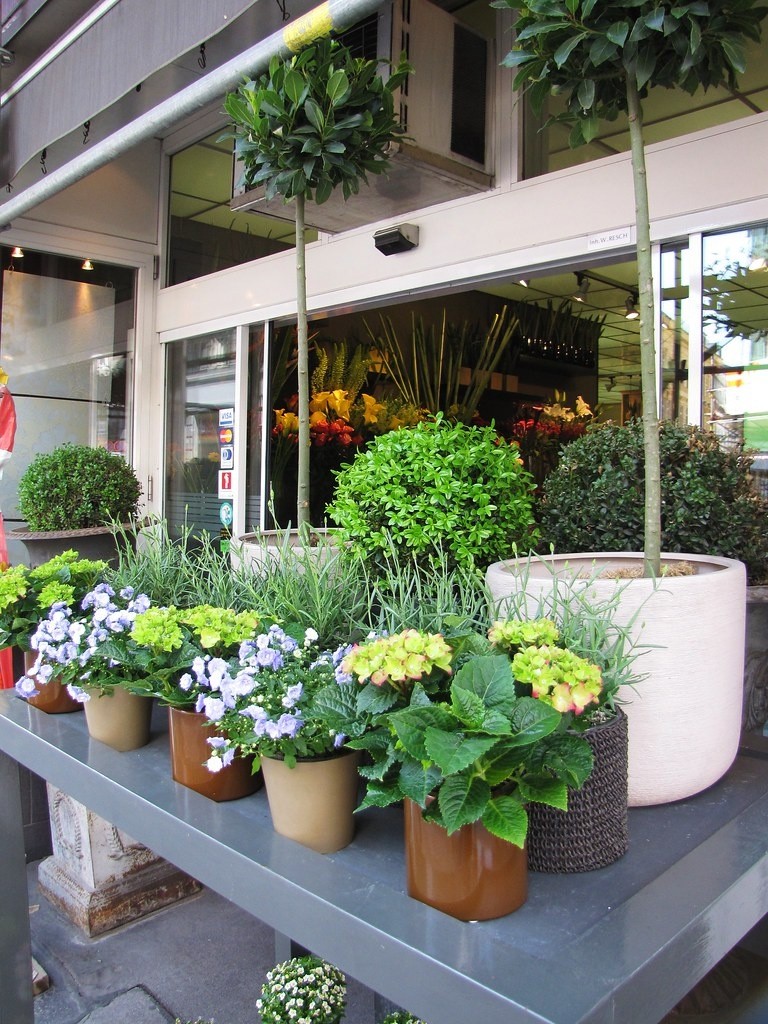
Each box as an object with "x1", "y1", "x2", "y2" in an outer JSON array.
[
  {"x1": 4, "y1": 439, "x2": 159, "y2": 570},
  {"x1": 225, "y1": 1, "x2": 753, "y2": 872}
]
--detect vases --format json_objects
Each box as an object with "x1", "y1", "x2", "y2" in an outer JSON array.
[
  {"x1": 168, "y1": 705, "x2": 265, "y2": 803},
  {"x1": 402, "y1": 794, "x2": 528, "y2": 922},
  {"x1": 82, "y1": 683, "x2": 153, "y2": 750},
  {"x1": 261, "y1": 750, "x2": 365, "y2": 854},
  {"x1": 23, "y1": 650, "x2": 83, "y2": 713}
]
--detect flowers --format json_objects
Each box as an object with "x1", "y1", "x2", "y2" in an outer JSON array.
[
  {"x1": 311, "y1": 615, "x2": 604, "y2": 850},
  {"x1": 268, "y1": 391, "x2": 437, "y2": 478},
  {"x1": 381, "y1": 1013, "x2": 427, "y2": 1024},
  {"x1": 179, "y1": 625, "x2": 392, "y2": 772},
  {"x1": 128, "y1": 606, "x2": 262, "y2": 711},
  {"x1": 255, "y1": 955, "x2": 349, "y2": 1024},
  {"x1": 511, "y1": 389, "x2": 603, "y2": 452},
  {"x1": 0, "y1": 549, "x2": 112, "y2": 652},
  {"x1": 14, "y1": 581, "x2": 169, "y2": 704}
]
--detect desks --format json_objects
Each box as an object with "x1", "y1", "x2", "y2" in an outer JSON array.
[{"x1": 0, "y1": 688, "x2": 767, "y2": 1024}]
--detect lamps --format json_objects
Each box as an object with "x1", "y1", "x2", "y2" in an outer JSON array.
[
  {"x1": 372, "y1": 223, "x2": 419, "y2": 257},
  {"x1": 572, "y1": 275, "x2": 589, "y2": 304},
  {"x1": 605, "y1": 376, "x2": 616, "y2": 391},
  {"x1": 11, "y1": 248, "x2": 24, "y2": 257},
  {"x1": 624, "y1": 294, "x2": 640, "y2": 319},
  {"x1": 82, "y1": 261, "x2": 94, "y2": 270}
]
